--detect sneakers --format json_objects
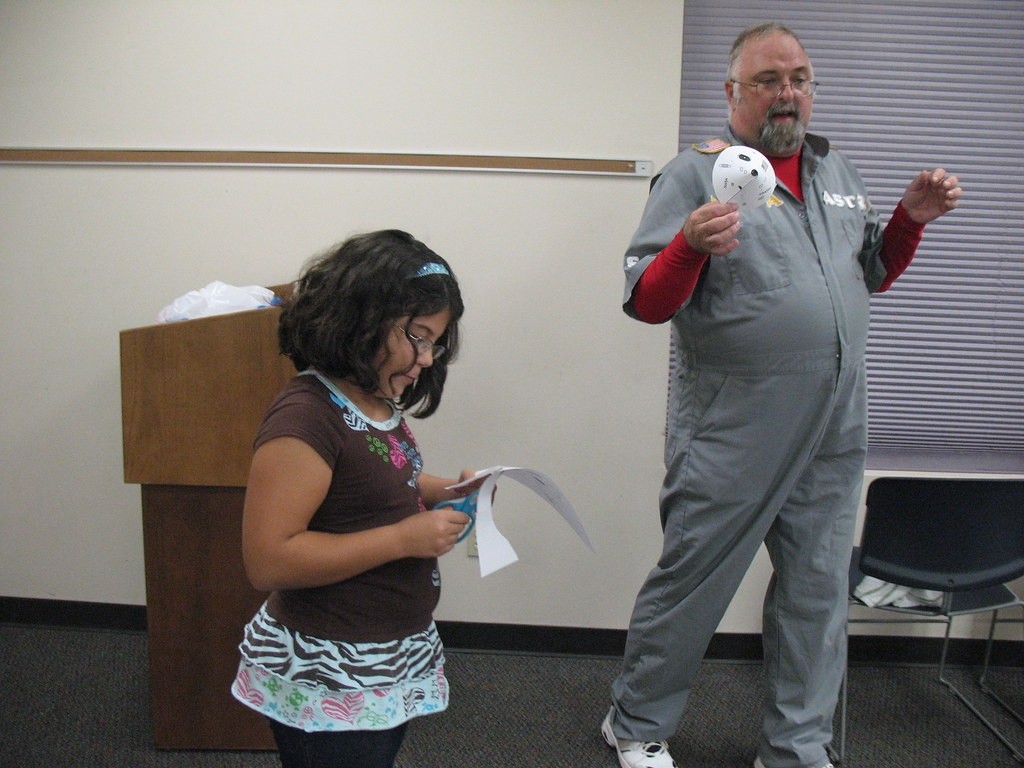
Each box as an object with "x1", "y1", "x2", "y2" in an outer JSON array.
[
  {"x1": 754, "y1": 755, "x2": 835, "y2": 768},
  {"x1": 602, "y1": 705, "x2": 676, "y2": 768}
]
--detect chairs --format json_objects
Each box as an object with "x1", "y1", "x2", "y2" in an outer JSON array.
[{"x1": 830, "y1": 472, "x2": 1024, "y2": 768}]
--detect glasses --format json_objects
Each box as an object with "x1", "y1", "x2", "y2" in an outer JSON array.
[
  {"x1": 389, "y1": 320, "x2": 446, "y2": 361},
  {"x1": 730, "y1": 77, "x2": 822, "y2": 99}
]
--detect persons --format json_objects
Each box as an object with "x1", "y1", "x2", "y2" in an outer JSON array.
[
  {"x1": 229, "y1": 229, "x2": 498, "y2": 768},
  {"x1": 600, "y1": 21, "x2": 963, "y2": 768}
]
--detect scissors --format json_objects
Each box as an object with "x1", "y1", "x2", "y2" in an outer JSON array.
[{"x1": 434, "y1": 483, "x2": 480, "y2": 543}]
